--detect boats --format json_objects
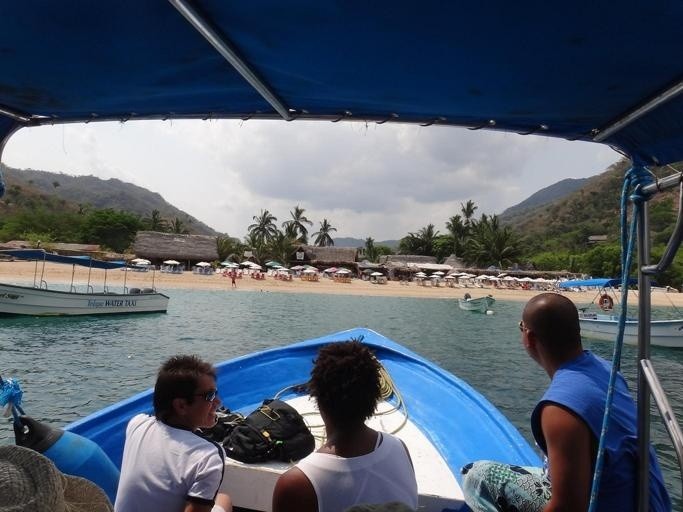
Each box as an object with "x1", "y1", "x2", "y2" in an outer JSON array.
[
  {"x1": 458, "y1": 296, "x2": 495, "y2": 315},
  {"x1": 0, "y1": 326, "x2": 552, "y2": 512},
  {"x1": 559, "y1": 276, "x2": 682, "y2": 349},
  {"x1": 0, "y1": 249, "x2": 170, "y2": 315}
]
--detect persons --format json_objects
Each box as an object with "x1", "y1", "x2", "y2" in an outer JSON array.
[
  {"x1": 461, "y1": 294, "x2": 674, "y2": 512},
  {"x1": 272, "y1": 334, "x2": 420, "y2": 511},
  {"x1": 112, "y1": 353, "x2": 233, "y2": 512},
  {"x1": 1, "y1": 442, "x2": 115, "y2": 511}
]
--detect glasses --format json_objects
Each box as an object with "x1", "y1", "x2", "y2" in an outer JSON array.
[
  {"x1": 519, "y1": 320, "x2": 526, "y2": 333},
  {"x1": 199, "y1": 387, "x2": 218, "y2": 402}
]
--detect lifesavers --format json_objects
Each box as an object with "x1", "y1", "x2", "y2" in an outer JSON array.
[{"x1": 599, "y1": 294, "x2": 613, "y2": 311}]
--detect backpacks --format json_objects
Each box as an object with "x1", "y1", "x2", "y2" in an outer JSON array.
[{"x1": 223, "y1": 399, "x2": 316, "y2": 464}]
